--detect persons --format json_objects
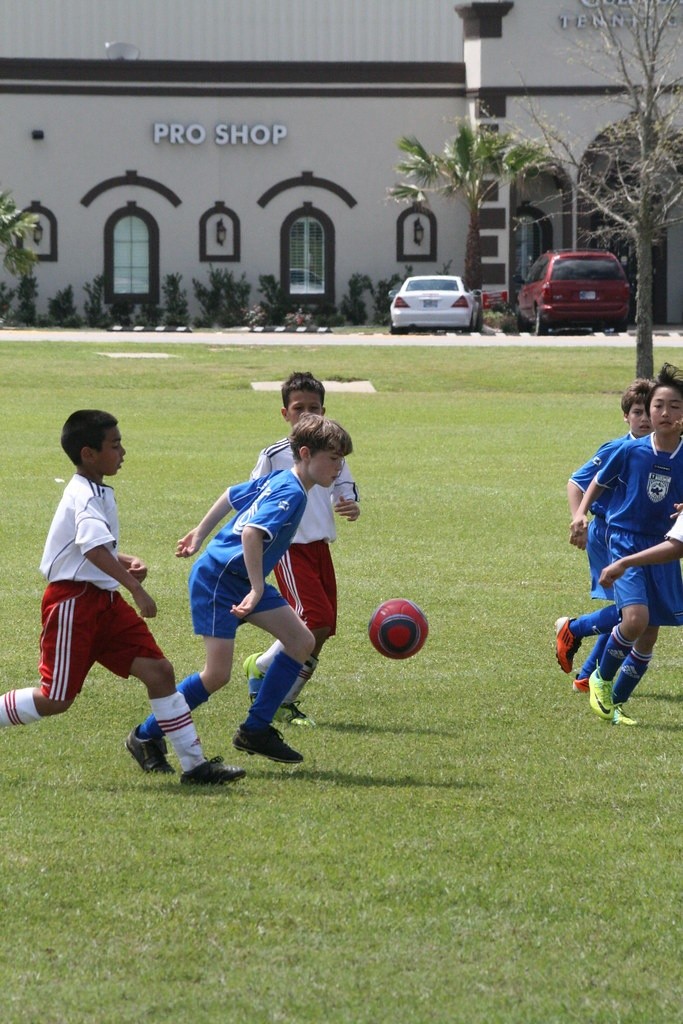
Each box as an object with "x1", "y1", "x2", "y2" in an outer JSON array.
[
  {"x1": 554, "y1": 363, "x2": 683, "y2": 726},
  {"x1": 242, "y1": 373, "x2": 361, "y2": 730},
  {"x1": 0, "y1": 409, "x2": 245, "y2": 784},
  {"x1": 125, "y1": 414, "x2": 354, "y2": 774}
]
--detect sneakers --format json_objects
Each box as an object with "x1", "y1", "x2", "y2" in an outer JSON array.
[
  {"x1": 555, "y1": 617, "x2": 583, "y2": 674},
  {"x1": 598, "y1": 703, "x2": 637, "y2": 726},
  {"x1": 180, "y1": 756, "x2": 246, "y2": 785},
  {"x1": 573, "y1": 674, "x2": 589, "y2": 693},
  {"x1": 274, "y1": 700, "x2": 317, "y2": 728},
  {"x1": 243, "y1": 652, "x2": 265, "y2": 704},
  {"x1": 589, "y1": 666, "x2": 614, "y2": 720},
  {"x1": 124, "y1": 724, "x2": 176, "y2": 775},
  {"x1": 232, "y1": 724, "x2": 303, "y2": 764}
]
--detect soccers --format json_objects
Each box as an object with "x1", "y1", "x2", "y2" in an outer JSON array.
[{"x1": 367, "y1": 599, "x2": 430, "y2": 660}]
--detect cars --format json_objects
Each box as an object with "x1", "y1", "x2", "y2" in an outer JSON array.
[{"x1": 388, "y1": 275, "x2": 483, "y2": 332}]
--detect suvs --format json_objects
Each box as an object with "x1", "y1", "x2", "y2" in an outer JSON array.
[{"x1": 511, "y1": 247, "x2": 632, "y2": 336}]
